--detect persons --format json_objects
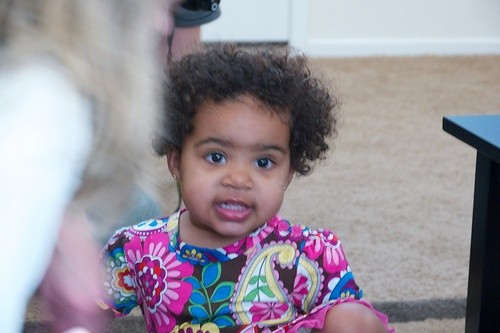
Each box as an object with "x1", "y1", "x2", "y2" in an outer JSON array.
[
  {"x1": 0, "y1": 1, "x2": 225, "y2": 333},
  {"x1": 40, "y1": 42, "x2": 395, "y2": 333}
]
